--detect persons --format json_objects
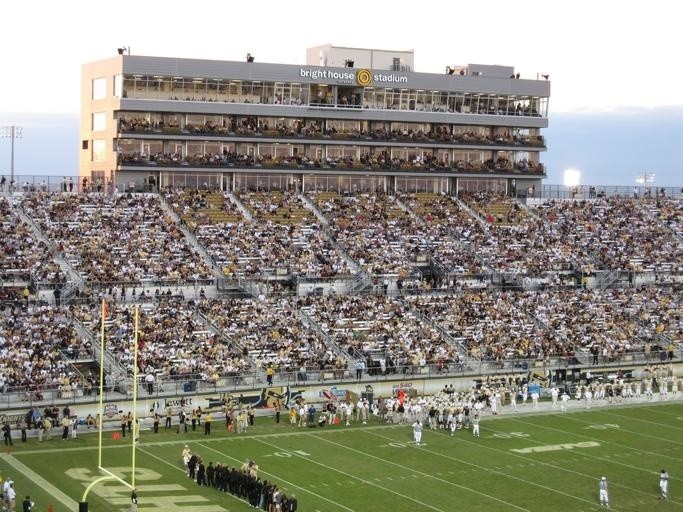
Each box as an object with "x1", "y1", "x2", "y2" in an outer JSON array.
[{"x1": 0, "y1": 88, "x2": 683, "y2": 512}]
